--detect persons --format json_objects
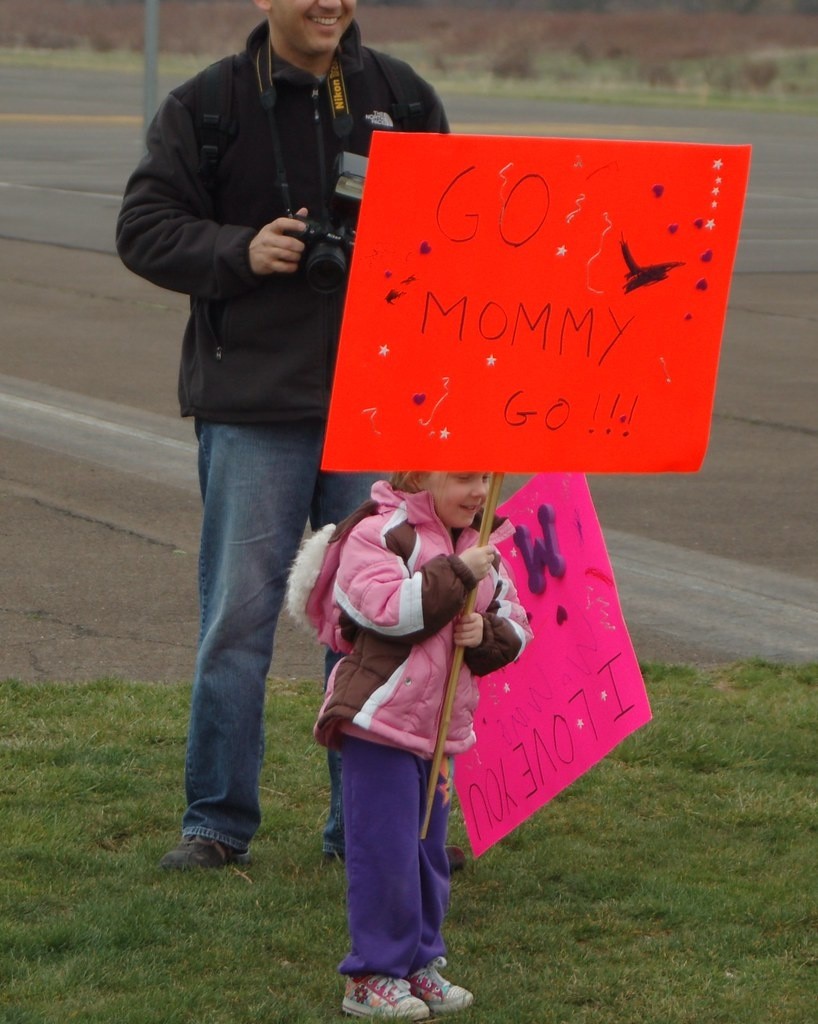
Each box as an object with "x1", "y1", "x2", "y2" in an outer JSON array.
[
  {"x1": 114, "y1": 0, "x2": 449, "y2": 867},
  {"x1": 287, "y1": 472, "x2": 534, "y2": 1020}
]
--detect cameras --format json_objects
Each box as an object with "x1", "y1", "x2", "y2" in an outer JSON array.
[{"x1": 281, "y1": 213, "x2": 356, "y2": 293}]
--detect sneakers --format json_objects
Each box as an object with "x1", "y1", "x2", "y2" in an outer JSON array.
[
  {"x1": 160, "y1": 831, "x2": 249, "y2": 870},
  {"x1": 445, "y1": 846, "x2": 464, "y2": 874},
  {"x1": 410, "y1": 957, "x2": 473, "y2": 1012},
  {"x1": 341, "y1": 970, "x2": 429, "y2": 1021}
]
